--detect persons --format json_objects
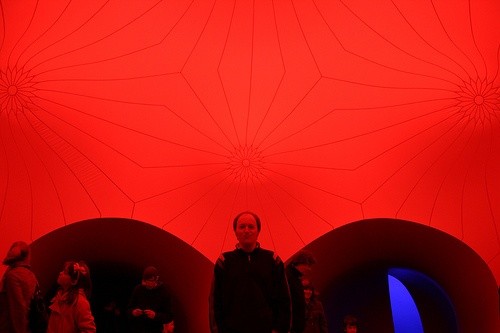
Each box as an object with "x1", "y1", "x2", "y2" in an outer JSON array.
[
  {"x1": 209, "y1": 211, "x2": 292, "y2": 333},
  {"x1": 286, "y1": 249, "x2": 330, "y2": 333},
  {"x1": 46, "y1": 260, "x2": 96, "y2": 333},
  {"x1": 127, "y1": 265, "x2": 173, "y2": 333},
  {"x1": 0, "y1": 242, "x2": 42, "y2": 333},
  {"x1": 341, "y1": 315, "x2": 360, "y2": 333}
]
